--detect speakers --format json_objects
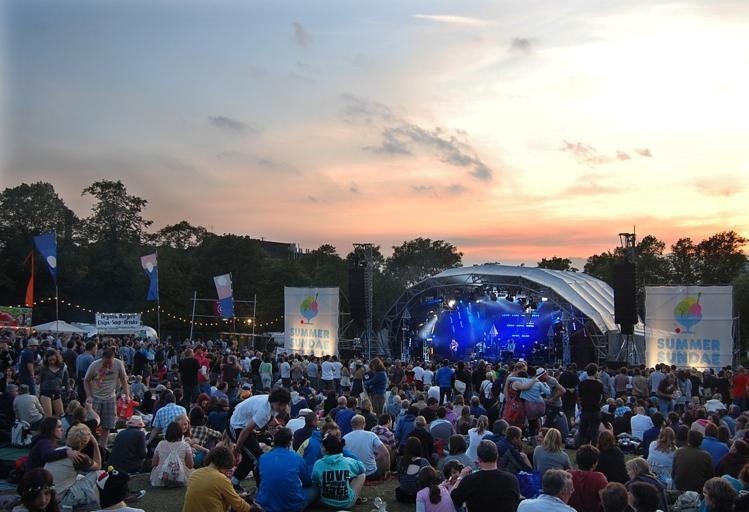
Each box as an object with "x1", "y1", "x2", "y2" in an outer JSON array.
[
  {"x1": 613, "y1": 262, "x2": 639, "y2": 324},
  {"x1": 349, "y1": 267, "x2": 374, "y2": 319}
]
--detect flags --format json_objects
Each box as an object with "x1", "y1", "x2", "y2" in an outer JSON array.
[
  {"x1": 213, "y1": 273, "x2": 233, "y2": 318},
  {"x1": 33, "y1": 231, "x2": 58, "y2": 286},
  {"x1": 139, "y1": 253, "x2": 159, "y2": 302}
]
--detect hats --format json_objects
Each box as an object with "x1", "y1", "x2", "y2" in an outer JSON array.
[
  {"x1": 154, "y1": 384, "x2": 166, "y2": 391},
  {"x1": 96, "y1": 465, "x2": 129, "y2": 508},
  {"x1": 126, "y1": 415, "x2": 146, "y2": 427},
  {"x1": 240, "y1": 384, "x2": 253, "y2": 400},
  {"x1": 536, "y1": 368, "x2": 546, "y2": 378},
  {"x1": 27, "y1": 339, "x2": 39, "y2": 347},
  {"x1": 129, "y1": 375, "x2": 142, "y2": 384},
  {"x1": 299, "y1": 408, "x2": 313, "y2": 415}
]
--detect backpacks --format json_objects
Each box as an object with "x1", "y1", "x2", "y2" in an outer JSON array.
[
  {"x1": 159, "y1": 442, "x2": 184, "y2": 482},
  {"x1": 12, "y1": 421, "x2": 32, "y2": 448}
]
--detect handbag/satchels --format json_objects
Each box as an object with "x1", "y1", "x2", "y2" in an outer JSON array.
[
  {"x1": 455, "y1": 380, "x2": 466, "y2": 394},
  {"x1": 518, "y1": 472, "x2": 540, "y2": 499},
  {"x1": 480, "y1": 390, "x2": 484, "y2": 404}
]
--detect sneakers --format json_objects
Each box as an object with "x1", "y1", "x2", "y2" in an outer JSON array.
[
  {"x1": 357, "y1": 497, "x2": 368, "y2": 504},
  {"x1": 136, "y1": 490, "x2": 146, "y2": 499}
]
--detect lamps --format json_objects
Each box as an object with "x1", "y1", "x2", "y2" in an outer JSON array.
[{"x1": 463, "y1": 283, "x2": 540, "y2": 315}]
